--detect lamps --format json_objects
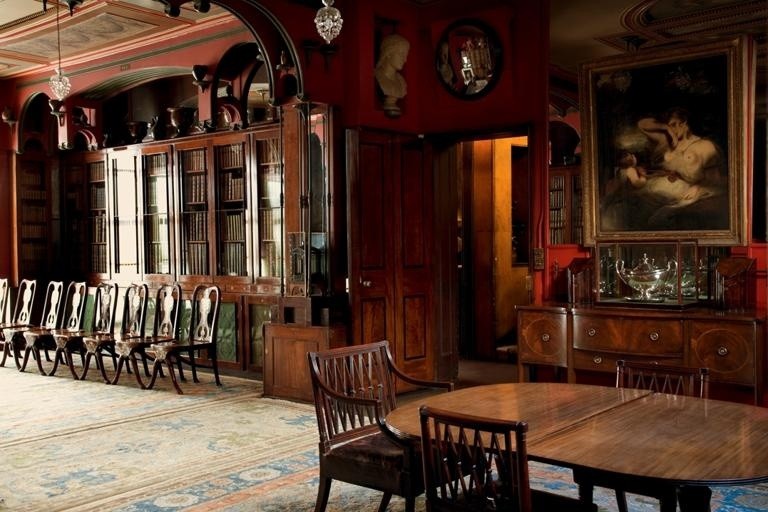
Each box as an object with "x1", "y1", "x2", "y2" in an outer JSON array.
[
  {"x1": 38, "y1": 0, "x2": 85, "y2": 101},
  {"x1": 314, "y1": 1, "x2": 344, "y2": 46}
]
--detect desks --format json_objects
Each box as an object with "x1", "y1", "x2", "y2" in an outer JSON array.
[{"x1": 377, "y1": 381, "x2": 768, "y2": 511}]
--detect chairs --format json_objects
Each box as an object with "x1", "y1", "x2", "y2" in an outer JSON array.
[
  {"x1": 145, "y1": 283, "x2": 221, "y2": 396},
  {"x1": 573, "y1": 357, "x2": 711, "y2": 512},
  {"x1": 0, "y1": 276, "x2": 87, "y2": 385},
  {"x1": 308, "y1": 339, "x2": 486, "y2": 512},
  {"x1": 111, "y1": 285, "x2": 185, "y2": 390},
  {"x1": 418, "y1": 404, "x2": 600, "y2": 512}
]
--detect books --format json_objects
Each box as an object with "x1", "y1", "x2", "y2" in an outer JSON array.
[
  {"x1": 18, "y1": 139, "x2": 284, "y2": 278},
  {"x1": 550, "y1": 172, "x2": 583, "y2": 246}
]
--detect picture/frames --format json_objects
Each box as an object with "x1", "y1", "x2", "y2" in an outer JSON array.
[{"x1": 574, "y1": 30, "x2": 759, "y2": 247}]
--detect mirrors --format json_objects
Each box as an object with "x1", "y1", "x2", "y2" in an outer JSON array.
[{"x1": 431, "y1": 17, "x2": 506, "y2": 101}]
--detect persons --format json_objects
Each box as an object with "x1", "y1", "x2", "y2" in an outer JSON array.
[
  {"x1": 604, "y1": 152, "x2": 647, "y2": 197},
  {"x1": 375, "y1": 31, "x2": 410, "y2": 103},
  {"x1": 635, "y1": 112, "x2": 720, "y2": 206}
]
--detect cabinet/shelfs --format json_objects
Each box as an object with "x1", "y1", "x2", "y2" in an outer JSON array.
[
  {"x1": 512, "y1": 298, "x2": 766, "y2": 406},
  {"x1": 7, "y1": 152, "x2": 68, "y2": 285}
]
